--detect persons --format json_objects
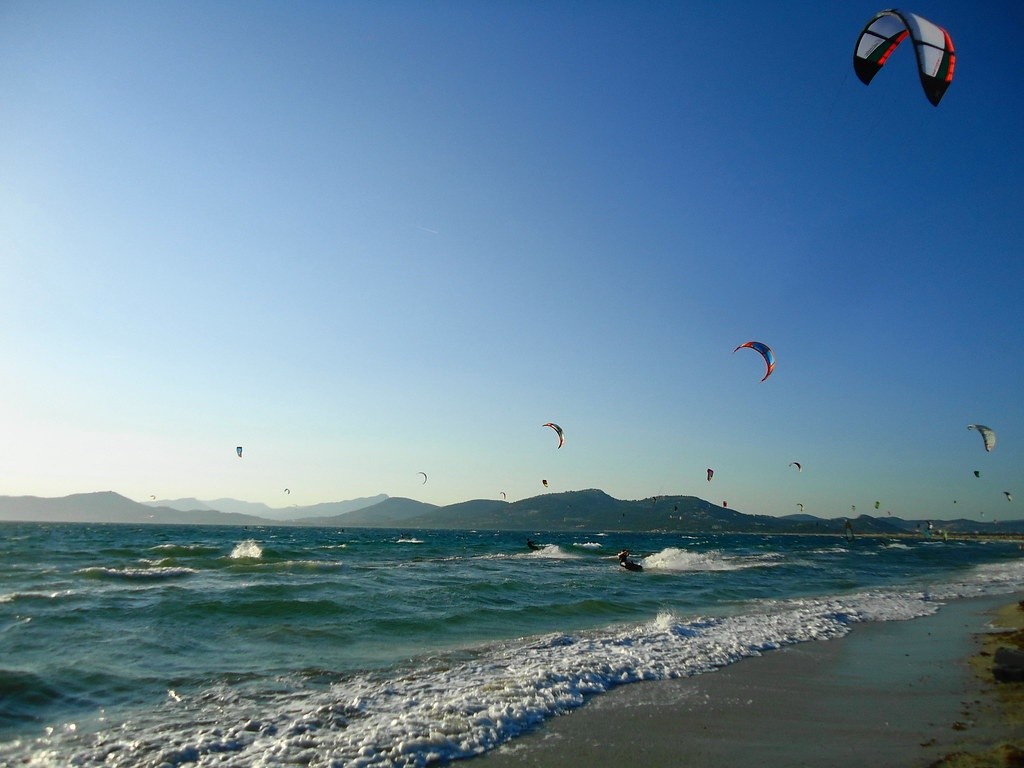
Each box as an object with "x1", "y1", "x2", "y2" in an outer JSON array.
[
  {"x1": 618, "y1": 549, "x2": 634, "y2": 566},
  {"x1": 527, "y1": 538, "x2": 538, "y2": 550},
  {"x1": 401, "y1": 533, "x2": 404, "y2": 539}
]
